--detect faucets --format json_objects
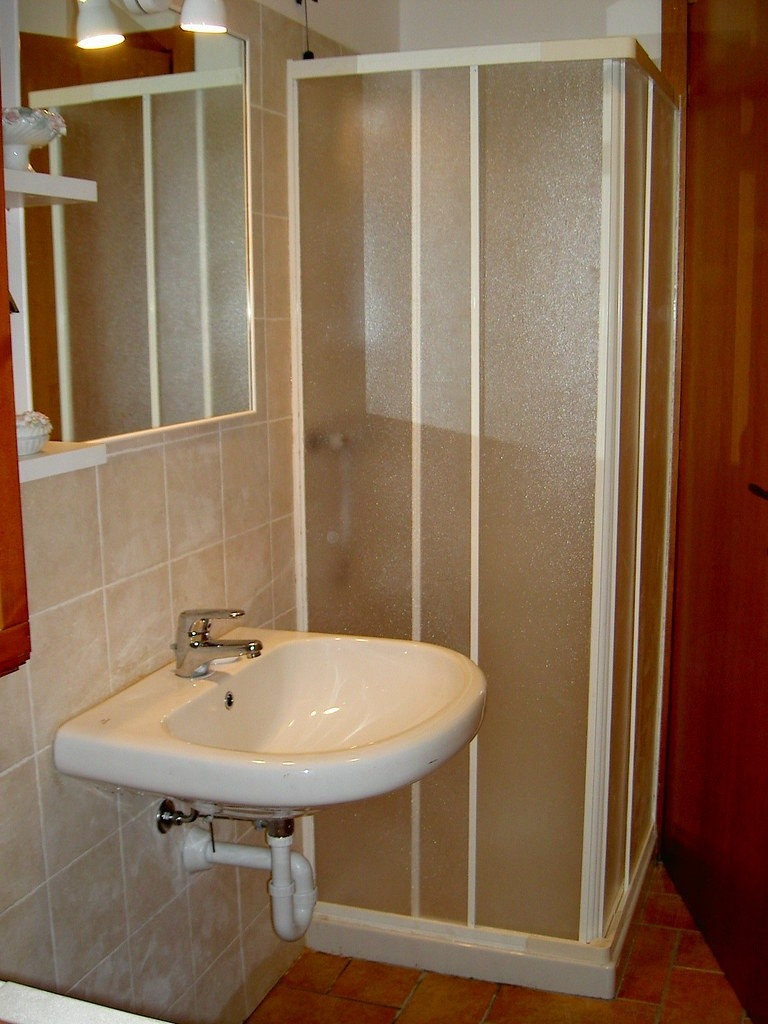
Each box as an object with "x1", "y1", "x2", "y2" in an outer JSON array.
[{"x1": 173, "y1": 608, "x2": 261, "y2": 678}]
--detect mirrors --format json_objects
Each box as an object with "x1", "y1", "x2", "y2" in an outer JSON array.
[{"x1": 0, "y1": 0, "x2": 257, "y2": 457}]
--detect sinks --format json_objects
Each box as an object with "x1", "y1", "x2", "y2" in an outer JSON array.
[{"x1": 154, "y1": 630, "x2": 491, "y2": 809}]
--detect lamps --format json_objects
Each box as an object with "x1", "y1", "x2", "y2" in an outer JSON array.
[
  {"x1": 180, "y1": 0, "x2": 229, "y2": 34},
  {"x1": 76, "y1": 0, "x2": 125, "y2": 50}
]
